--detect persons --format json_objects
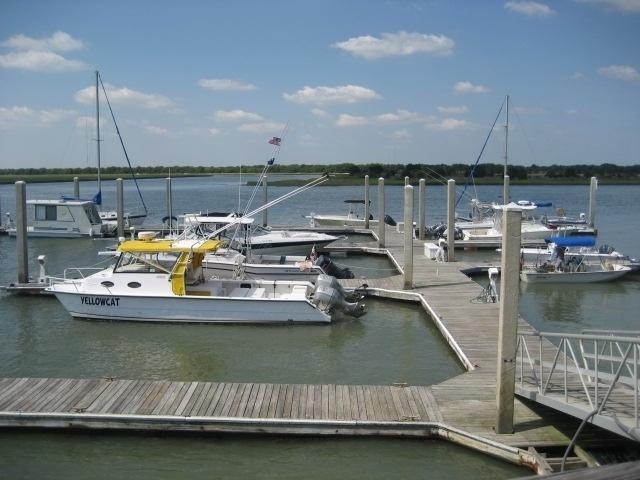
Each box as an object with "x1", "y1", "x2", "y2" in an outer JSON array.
[
  {"x1": 435, "y1": 233, "x2": 447, "y2": 262},
  {"x1": 548, "y1": 245, "x2": 569, "y2": 272}
]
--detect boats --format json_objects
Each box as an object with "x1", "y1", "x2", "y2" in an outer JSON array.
[
  {"x1": 494, "y1": 235, "x2": 639, "y2": 284},
  {"x1": 41, "y1": 171, "x2": 371, "y2": 326},
  {"x1": 305, "y1": 205, "x2": 418, "y2": 227},
  {"x1": 441, "y1": 93, "x2": 598, "y2": 249},
  {"x1": 6, "y1": 199, "x2": 108, "y2": 237},
  {"x1": 96, "y1": 119, "x2": 357, "y2": 284}
]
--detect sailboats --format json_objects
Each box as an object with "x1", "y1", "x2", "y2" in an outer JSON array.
[{"x1": 4, "y1": 67, "x2": 148, "y2": 227}]
